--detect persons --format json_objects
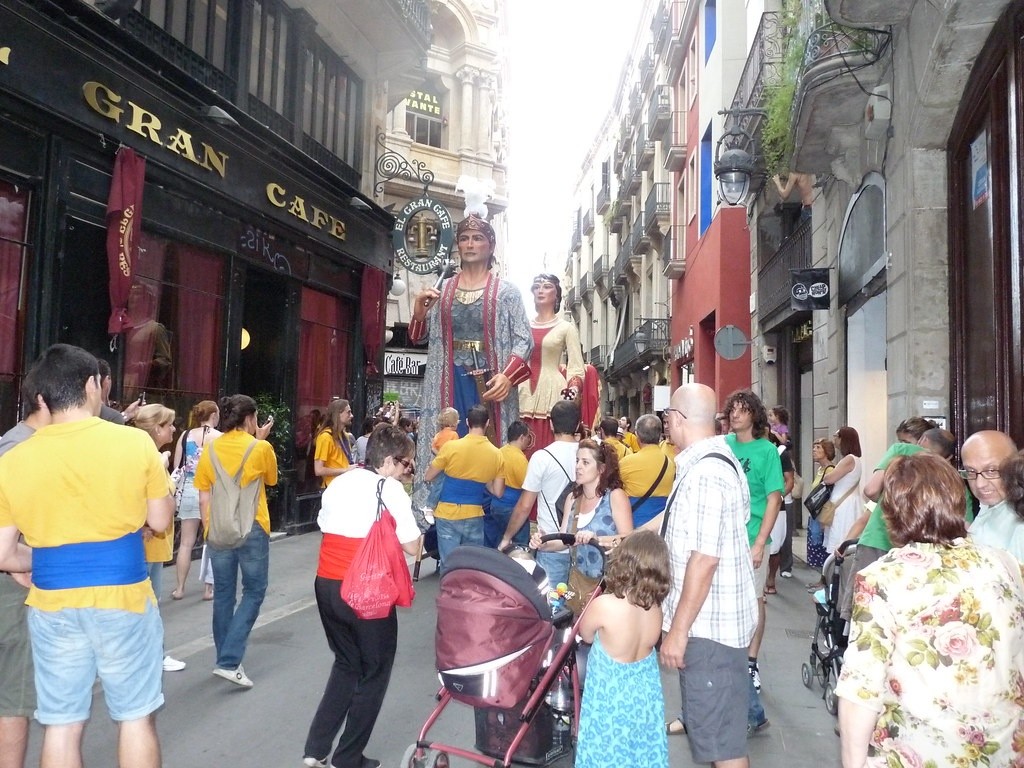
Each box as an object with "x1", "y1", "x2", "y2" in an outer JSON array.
[
  {"x1": 0, "y1": 343, "x2": 1024, "y2": 768},
  {"x1": 516, "y1": 272, "x2": 586, "y2": 522},
  {"x1": 122, "y1": 282, "x2": 175, "y2": 406},
  {"x1": 408, "y1": 216, "x2": 535, "y2": 531}
]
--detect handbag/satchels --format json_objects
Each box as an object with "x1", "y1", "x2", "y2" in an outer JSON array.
[
  {"x1": 803, "y1": 478, "x2": 835, "y2": 521},
  {"x1": 563, "y1": 567, "x2": 602, "y2": 617},
  {"x1": 208, "y1": 478, "x2": 262, "y2": 548},
  {"x1": 169, "y1": 430, "x2": 190, "y2": 516},
  {"x1": 340, "y1": 479, "x2": 415, "y2": 620},
  {"x1": 816, "y1": 500, "x2": 836, "y2": 526}
]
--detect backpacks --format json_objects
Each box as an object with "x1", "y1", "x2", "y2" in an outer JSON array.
[{"x1": 538, "y1": 448, "x2": 577, "y2": 529}]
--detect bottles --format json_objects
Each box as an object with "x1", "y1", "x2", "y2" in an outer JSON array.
[{"x1": 550, "y1": 675, "x2": 571, "y2": 753}]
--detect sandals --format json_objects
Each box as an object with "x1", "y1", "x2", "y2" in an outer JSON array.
[
  {"x1": 666, "y1": 720, "x2": 687, "y2": 735},
  {"x1": 746, "y1": 719, "x2": 769, "y2": 735}
]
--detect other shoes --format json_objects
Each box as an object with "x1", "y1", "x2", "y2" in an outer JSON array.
[
  {"x1": 172, "y1": 588, "x2": 182, "y2": 600},
  {"x1": 331, "y1": 752, "x2": 381, "y2": 768},
  {"x1": 781, "y1": 572, "x2": 792, "y2": 578},
  {"x1": 805, "y1": 583, "x2": 825, "y2": 594},
  {"x1": 205, "y1": 591, "x2": 214, "y2": 600},
  {"x1": 747, "y1": 661, "x2": 761, "y2": 694},
  {"x1": 765, "y1": 584, "x2": 777, "y2": 594},
  {"x1": 303, "y1": 756, "x2": 330, "y2": 767}
]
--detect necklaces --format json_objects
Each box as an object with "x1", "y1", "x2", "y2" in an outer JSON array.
[{"x1": 582, "y1": 489, "x2": 596, "y2": 499}]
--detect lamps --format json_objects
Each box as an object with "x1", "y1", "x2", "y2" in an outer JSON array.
[
  {"x1": 389, "y1": 269, "x2": 405, "y2": 298},
  {"x1": 350, "y1": 198, "x2": 372, "y2": 214},
  {"x1": 714, "y1": 105, "x2": 778, "y2": 206},
  {"x1": 633, "y1": 315, "x2": 670, "y2": 354},
  {"x1": 201, "y1": 107, "x2": 240, "y2": 129}
]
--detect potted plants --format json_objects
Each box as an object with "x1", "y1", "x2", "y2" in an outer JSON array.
[{"x1": 253, "y1": 393, "x2": 297, "y2": 526}]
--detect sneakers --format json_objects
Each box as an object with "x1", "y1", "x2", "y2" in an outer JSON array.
[
  {"x1": 213, "y1": 664, "x2": 254, "y2": 687},
  {"x1": 163, "y1": 656, "x2": 187, "y2": 672}
]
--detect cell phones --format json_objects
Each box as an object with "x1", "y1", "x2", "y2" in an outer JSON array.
[{"x1": 137, "y1": 394, "x2": 144, "y2": 408}]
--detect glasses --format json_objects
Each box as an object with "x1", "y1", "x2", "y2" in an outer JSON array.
[
  {"x1": 664, "y1": 407, "x2": 687, "y2": 420},
  {"x1": 394, "y1": 457, "x2": 409, "y2": 469},
  {"x1": 958, "y1": 468, "x2": 1000, "y2": 480}
]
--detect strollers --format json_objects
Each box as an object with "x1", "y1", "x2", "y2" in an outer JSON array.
[
  {"x1": 399, "y1": 535, "x2": 610, "y2": 768},
  {"x1": 801, "y1": 536, "x2": 859, "y2": 716}
]
